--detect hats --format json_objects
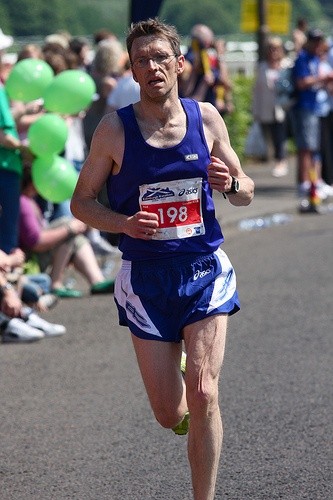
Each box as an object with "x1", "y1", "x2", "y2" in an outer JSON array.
[
  {"x1": 0, "y1": 30, "x2": 90, "y2": 63},
  {"x1": 307, "y1": 27, "x2": 328, "y2": 39}
]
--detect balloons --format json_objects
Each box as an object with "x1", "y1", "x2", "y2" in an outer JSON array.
[
  {"x1": 30, "y1": 153, "x2": 79, "y2": 203},
  {"x1": 26, "y1": 112, "x2": 70, "y2": 158},
  {"x1": 4, "y1": 57, "x2": 55, "y2": 105},
  {"x1": 43, "y1": 68, "x2": 97, "y2": 116}
]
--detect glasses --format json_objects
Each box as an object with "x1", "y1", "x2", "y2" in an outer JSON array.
[{"x1": 131, "y1": 53, "x2": 177, "y2": 68}]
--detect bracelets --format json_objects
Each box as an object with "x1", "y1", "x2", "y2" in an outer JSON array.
[{"x1": 0, "y1": 282, "x2": 12, "y2": 292}]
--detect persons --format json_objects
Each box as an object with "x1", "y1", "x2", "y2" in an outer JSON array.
[
  {"x1": 0, "y1": 21, "x2": 235, "y2": 345},
  {"x1": 70, "y1": 18, "x2": 255, "y2": 500},
  {"x1": 250, "y1": 17, "x2": 333, "y2": 216}
]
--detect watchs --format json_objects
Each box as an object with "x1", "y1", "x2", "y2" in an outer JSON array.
[{"x1": 222, "y1": 175, "x2": 241, "y2": 199}]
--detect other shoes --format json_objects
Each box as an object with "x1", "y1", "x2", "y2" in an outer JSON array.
[
  {"x1": 90, "y1": 279, "x2": 115, "y2": 294},
  {"x1": 35, "y1": 293, "x2": 58, "y2": 313},
  {"x1": 298, "y1": 179, "x2": 333, "y2": 202},
  {"x1": 273, "y1": 160, "x2": 287, "y2": 178},
  {"x1": 51, "y1": 285, "x2": 83, "y2": 299}
]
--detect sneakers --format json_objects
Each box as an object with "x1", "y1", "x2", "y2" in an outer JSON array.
[
  {"x1": 168, "y1": 352, "x2": 191, "y2": 436},
  {"x1": 2, "y1": 317, "x2": 45, "y2": 343},
  {"x1": 22, "y1": 314, "x2": 67, "y2": 338}
]
